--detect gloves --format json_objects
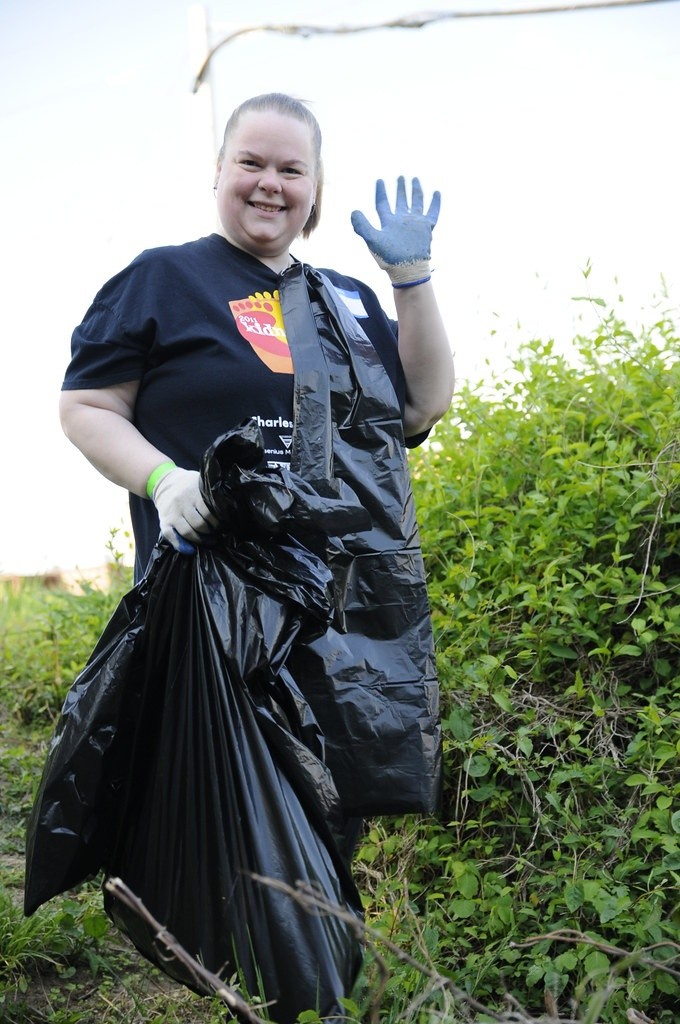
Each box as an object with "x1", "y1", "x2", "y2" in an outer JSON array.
[
  {"x1": 350, "y1": 175, "x2": 440, "y2": 287},
  {"x1": 146, "y1": 463, "x2": 218, "y2": 554}
]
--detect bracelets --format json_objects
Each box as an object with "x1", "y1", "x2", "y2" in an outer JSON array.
[{"x1": 147, "y1": 462, "x2": 176, "y2": 494}]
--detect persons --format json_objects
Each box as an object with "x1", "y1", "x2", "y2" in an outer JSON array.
[{"x1": 59, "y1": 93, "x2": 455, "y2": 1023}]
